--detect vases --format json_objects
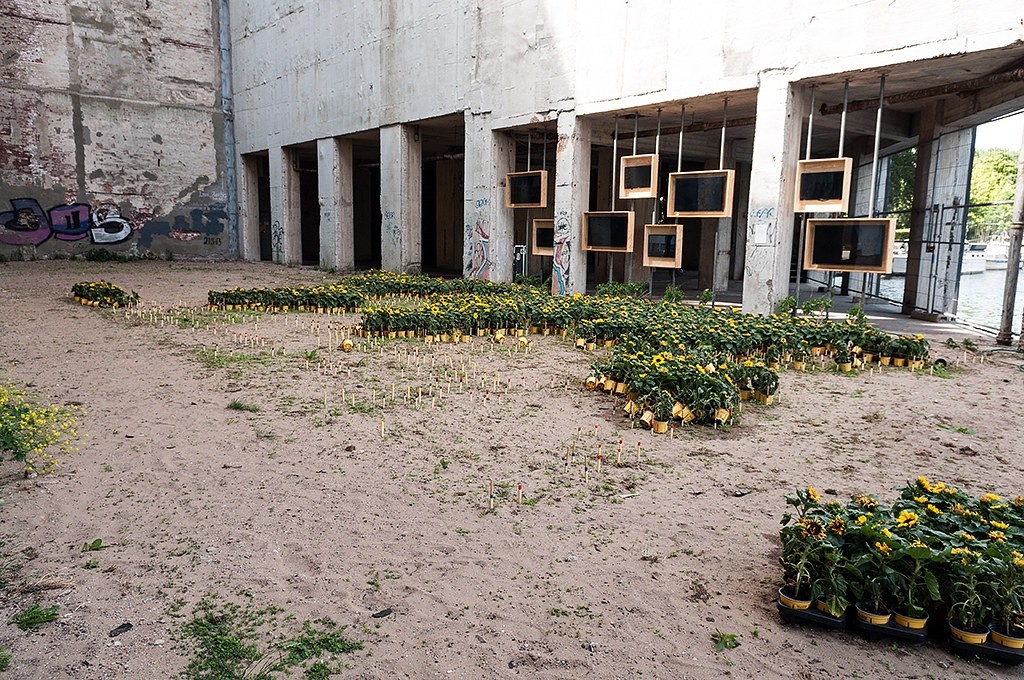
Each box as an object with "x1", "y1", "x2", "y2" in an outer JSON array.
[
  {"x1": 739, "y1": 347, "x2": 924, "y2": 406},
  {"x1": 777, "y1": 584, "x2": 1024, "y2": 649},
  {"x1": 586, "y1": 375, "x2": 729, "y2": 434}
]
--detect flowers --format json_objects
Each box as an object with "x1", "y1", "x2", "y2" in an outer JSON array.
[
  {"x1": 207, "y1": 269, "x2": 574, "y2": 333},
  {"x1": 585, "y1": 357, "x2": 779, "y2": 417},
  {"x1": 571, "y1": 293, "x2": 928, "y2": 361},
  {"x1": 777, "y1": 475, "x2": 1024, "y2": 626},
  {"x1": 71, "y1": 277, "x2": 142, "y2": 308}
]
